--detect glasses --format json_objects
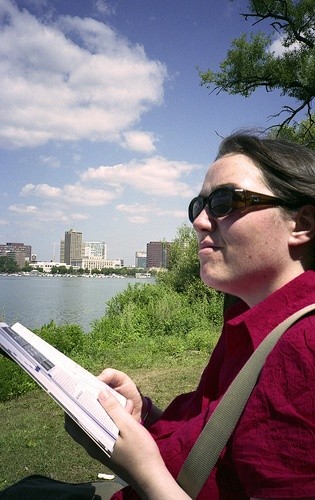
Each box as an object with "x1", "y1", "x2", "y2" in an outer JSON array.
[{"x1": 187, "y1": 187, "x2": 295, "y2": 223}]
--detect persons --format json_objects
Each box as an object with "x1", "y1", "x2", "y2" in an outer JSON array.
[{"x1": 64, "y1": 129, "x2": 315, "y2": 500}]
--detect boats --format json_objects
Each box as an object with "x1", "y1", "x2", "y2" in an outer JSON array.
[{"x1": 3, "y1": 269, "x2": 152, "y2": 279}]
